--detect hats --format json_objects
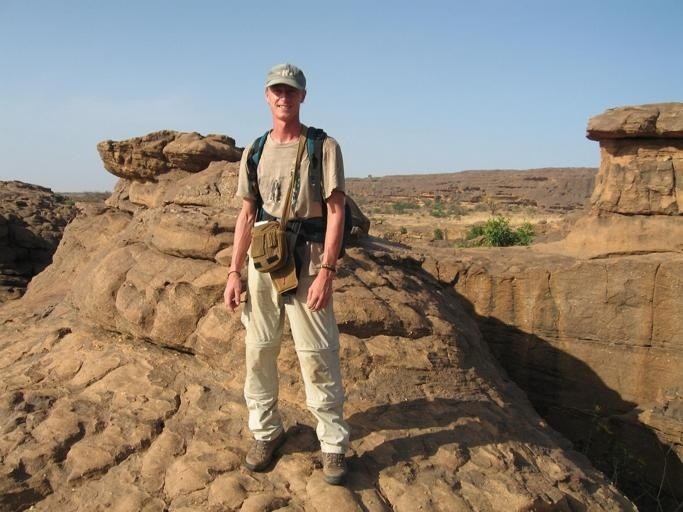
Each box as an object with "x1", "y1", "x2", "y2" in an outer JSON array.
[{"x1": 265, "y1": 63, "x2": 306, "y2": 93}]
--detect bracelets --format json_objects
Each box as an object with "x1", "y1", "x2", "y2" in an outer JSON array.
[
  {"x1": 226, "y1": 270, "x2": 242, "y2": 279},
  {"x1": 317, "y1": 261, "x2": 337, "y2": 273}
]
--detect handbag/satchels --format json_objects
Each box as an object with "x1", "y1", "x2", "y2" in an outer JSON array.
[{"x1": 250, "y1": 223, "x2": 288, "y2": 274}]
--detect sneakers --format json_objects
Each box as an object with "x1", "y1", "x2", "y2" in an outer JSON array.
[
  {"x1": 321, "y1": 452, "x2": 347, "y2": 485},
  {"x1": 245, "y1": 428, "x2": 287, "y2": 472}
]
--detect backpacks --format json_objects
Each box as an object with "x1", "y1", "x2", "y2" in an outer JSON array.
[{"x1": 247, "y1": 127, "x2": 371, "y2": 249}]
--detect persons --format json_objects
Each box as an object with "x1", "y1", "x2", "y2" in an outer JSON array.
[{"x1": 220, "y1": 63, "x2": 351, "y2": 485}]
